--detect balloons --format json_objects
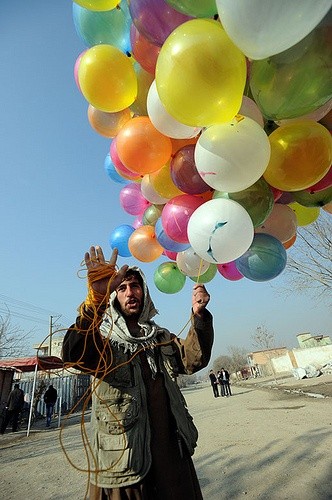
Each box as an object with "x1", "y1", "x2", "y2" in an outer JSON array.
[
  {"x1": 153, "y1": 261, "x2": 186, "y2": 295},
  {"x1": 72, "y1": 0, "x2": 332, "y2": 139},
  {"x1": 155, "y1": 193, "x2": 217, "y2": 284},
  {"x1": 186, "y1": 198, "x2": 254, "y2": 264},
  {"x1": 216, "y1": 232, "x2": 287, "y2": 282},
  {"x1": 104, "y1": 117, "x2": 211, "y2": 230},
  {"x1": 154, "y1": 19, "x2": 248, "y2": 127},
  {"x1": 109, "y1": 224, "x2": 164, "y2": 263},
  {"x1": 171, "y1": 114, "x2": 332, "y2": 249}
]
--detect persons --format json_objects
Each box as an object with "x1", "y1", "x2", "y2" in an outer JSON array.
[
  {"x1": 43, "y1": 385, "x2": 57, "y2": 427},
  {"x1": 209, "y1": 369, "x2": 221, "y2": 398},
  {"x1": 5, "y1": 384, "x2": 24, "y2": 432},
  {"x1": 216, "y1": 367, "x2": 233, "y2": 397},
  {"x1": 61, "y1": 245, "x2": 214, "y2": 500}
]
